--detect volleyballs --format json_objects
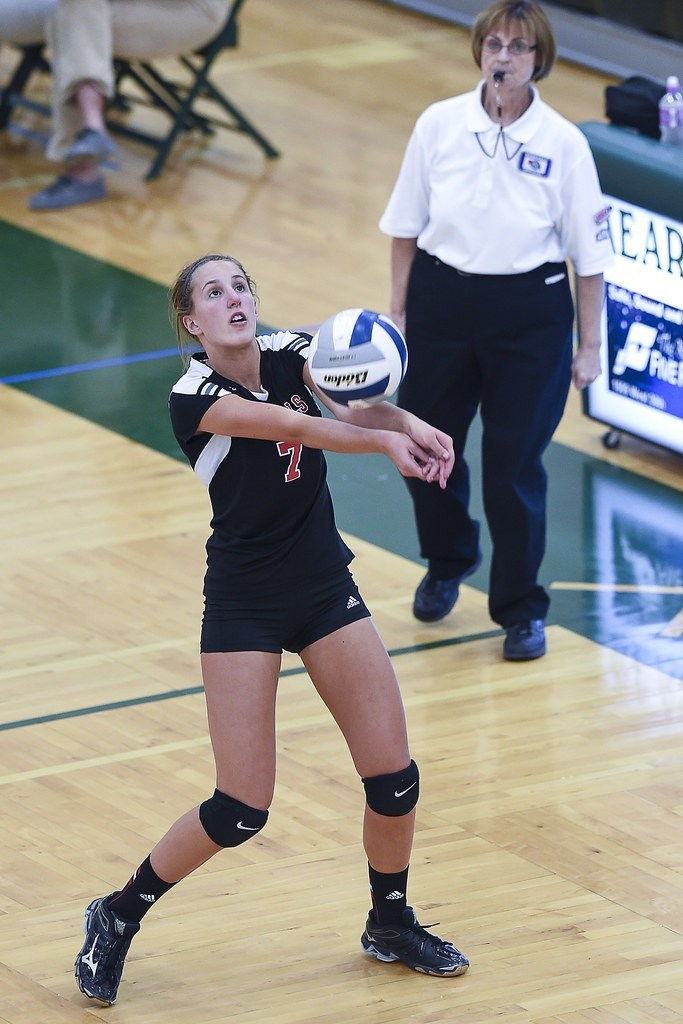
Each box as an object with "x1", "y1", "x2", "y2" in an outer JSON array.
[{"x1": 308, "y1": 308, "x2": 408, "y2": 409}]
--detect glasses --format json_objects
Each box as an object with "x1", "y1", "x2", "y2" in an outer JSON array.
[{"x1": 481, "y1": 36, "x2": 537, "y2": 56}]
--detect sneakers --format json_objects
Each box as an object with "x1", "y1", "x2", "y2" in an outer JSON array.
[
  {"x1": 359, "y1": 908, "x2": 470, "y2": 980},
  {"x1": 74, "y1": 894, "x2": 140, "y2": 1007}
]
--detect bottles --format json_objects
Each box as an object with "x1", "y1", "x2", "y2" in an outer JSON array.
[{"x1": 655, "y1": 76, "x2": 683, "y2": 147}]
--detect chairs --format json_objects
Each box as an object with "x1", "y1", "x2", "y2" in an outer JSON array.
[{"x1": 0, "y1": 0, "x2": 278, "y2": 182}]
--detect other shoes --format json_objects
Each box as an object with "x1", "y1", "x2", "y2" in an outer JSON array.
[
  {"x1": 413, "y1": 547, "x2": 484, "y2": 623},
  {"x1": 30, "y1": 168, "x2": 109, "y2": 212},
  {"x1": 66, "y1": 127, "x2": 115, "y2": 167},
  {"x1": 503, "y1": 620, "x2": 548, "y2": 661}
]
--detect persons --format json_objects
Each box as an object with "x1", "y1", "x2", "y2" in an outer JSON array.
[
  {"x1": 28, "y1": 0, "x2": 235, "y2": 212},
  {"x1": 378, "y1": 0, "x2": 612, "y2": 661},
  {"x1": 73, "y1": 253, "x2": 468, "y2": 1007}
]
